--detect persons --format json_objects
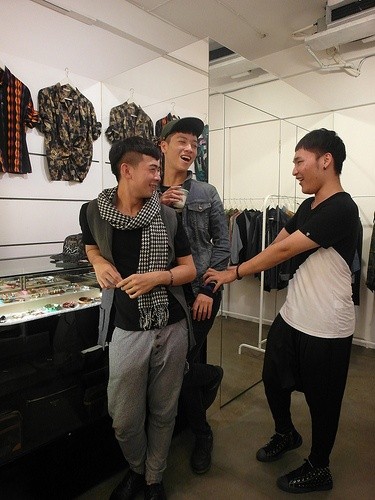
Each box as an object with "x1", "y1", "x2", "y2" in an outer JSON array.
[
  {"x1": 78, "y1": 136, "x2": 197, "y2": 500},
  {"x1": 203, "y1": 128, "x2": 359, "y2": 494},
  {"x1": 158, "y1": 117, "x2": 232, "y2": 475}
]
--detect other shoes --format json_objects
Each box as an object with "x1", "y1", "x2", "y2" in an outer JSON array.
[
  {"x1": 197, "y1": 366, "x2": 223, "y2": 409},
  {"x1": 191, "y1": 430, "x2": 213, "y2": 475}
]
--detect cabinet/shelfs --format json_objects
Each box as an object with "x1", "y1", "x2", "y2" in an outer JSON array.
[{"x1": 0, "y1": 305, "x2": 207, "y2": 467}]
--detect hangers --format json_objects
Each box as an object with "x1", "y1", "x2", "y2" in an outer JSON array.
[
  {"x1": 0, "y1": 54, "x2": 29, "y2": 90},
  {"x1": 157, "y1": 103, "x2": 208, "y2": 136},
  {"x1": 224, "y1": 197, "x2": 295, "y2": 220},
  {"x1": 39, "y1": 67, "x2": 92, "y2": 103},
  {"x1": 110, "y1": 88, "x2": 153, "y2": 123}
]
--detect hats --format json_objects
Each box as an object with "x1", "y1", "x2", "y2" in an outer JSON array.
[
  {"x1": 161, "y1": 118, "x2": 203, "y2": 140},
  {"x1": 50, "y1": 234, "x2": 86, "y2": 263}
]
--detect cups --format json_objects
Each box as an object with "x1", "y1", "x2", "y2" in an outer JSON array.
[{"x1": 172, "y1": 188, "x2": 190, "y2": 209}]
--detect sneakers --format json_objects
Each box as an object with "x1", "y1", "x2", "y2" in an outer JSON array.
[
  {"x1": 108, "y1": 468, "x2": 144, "y2": 500},
  {"x1": 256, "y1": 428, "x2": 302, "y2": 462},
  {"x1": 144, "y1": 480, "x2": 166, "y2": 500},
  {"x1": 278, "y1": 457, "x2": 333, "y2": 492}
]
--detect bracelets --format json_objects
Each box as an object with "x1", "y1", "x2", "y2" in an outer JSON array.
[
  {"x1": 236, "y1": 264, "x2": 242, "y2": 280},
  {"x1": 169, "y1": 270, "x2": 173, "y2": 286}
]
